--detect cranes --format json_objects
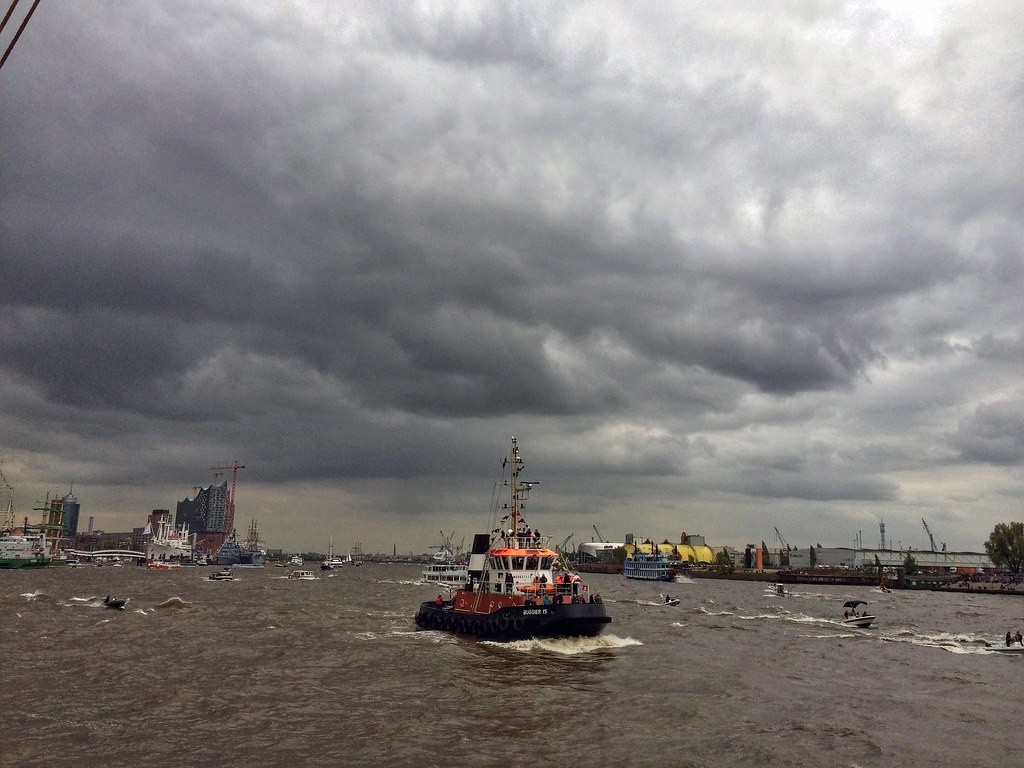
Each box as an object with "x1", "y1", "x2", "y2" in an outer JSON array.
[{"x1": 208, "y1": 459, "x2": 246, "y2": 536}]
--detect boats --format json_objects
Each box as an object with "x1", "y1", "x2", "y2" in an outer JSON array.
[
  {"x1": 843, "y1": 600, "x2": 876, "y2": 628},
  {"x1": 661, "y1": 599, "x2": 681, "y2": 606},
  {"x1": 983, "y1": 635, "x2": 1024, "y2": 651},
  {"x1": 291, "y1": 555, "x2": 304, "y2": 566},
  {"x1": 288, "y1": 570, "x2": 316, "y2": 581},
  {"x1": 623, "y1": 541, "x2": 687, "y2": 580},
  {"x1": 104, "y1": 600, "x2": 125, "y2": 607},
  {"x1": 420, "y1": 529, "x2": 473, "y2": 585},
  {"x1": 321, "y1": 556, "x2": 343, "y2": 571},
  {"x1": 904, "y1": 575, "x2": 950, "y2": 591},
  {"x1": 66, "y1": 559, "x2": 80, "y2": 568},
  {"x1": 274, "y1": 561, "x2": 291, "y2": 567},
  {"x1": 935, "y1": 573, "x2": 1024, "y2": 594},
  {"x1": 209, "y1": 567, "x2": 235, "y2": 580},
  {"x1": 674, "y1": 573, "x2": 696, "y2": 584},
  {"x1": 870, "y1": 587, "x2": 893, "y2": 593},
  {"x1": 771, "y1": 583, "x2": 791, "y2": 598}
]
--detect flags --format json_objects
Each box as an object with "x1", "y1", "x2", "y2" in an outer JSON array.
[
  {"x1": 511, "y1": 510, "x2": 581, "y2": 584},
  {"x1": 143, "y1": 522, "x2": 151, "y2": 534}
]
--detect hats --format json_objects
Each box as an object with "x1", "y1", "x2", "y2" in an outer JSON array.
[{"x1": 439, "y1": 593, "x2": 441, "y2": 595}]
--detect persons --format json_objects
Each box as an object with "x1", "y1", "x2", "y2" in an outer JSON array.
[
  {"x1": 590, "y1": 591, "x2": 602, "y2": 603},
  {"x1": 666, "y1": 595, "x2": 670, "y2": 602},
  {"x1": 529, "y1": 592, "x2": 564, "y2": 605},
  {"x1": 104, "y1": 595, "x2": 110, "y2": 603},
  {"x1": 1005, "y1": 631, "x2": 1024, "y2": 647},
  {"x1": 435, "y1": 593, "x2": 443, "y2": 608},
  {"x1": 529, "y1": 558, "x2": 533, "y2": 567},
  {"x1": 533, "y1": 529, "x2": 540, "y2": 547},
  {"x1": 571, "y1": 573, "x2": 580, "y2": 595},
  {"x1": 561, "y1": 573, "x2": 570, "y2": 595},
  {"x1": 533, "y1": 559, "x2": 536, "y2": 567},
  {"x1": 844, "y1": 610, "x2": 871, "y2": 619},
  {"x1": 505, "y1": 572, "x2": 513, "y2": 592},
  {"x1": 526, "y1": 529, "x2": 532, "y2": 546},
  {"x1": 571, "y1": 593, "x2": 586, "y2": 603},
  {"x1": 539, "y1": 574, "x2": 547, "y2": 594}
]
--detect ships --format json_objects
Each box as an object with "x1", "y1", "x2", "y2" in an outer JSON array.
[
  {"x1": 144, "y1": 514, "x2": 213, "y2": 569},
  {"x1": 206, "y1": 529, "x2": 267, "y2": 569},
  {"x1": 0, "y1": 528, "x2": 51, "y2": 568}
]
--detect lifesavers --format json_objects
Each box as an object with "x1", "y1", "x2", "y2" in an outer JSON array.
[
  {"x1": 511, "y1": 602, "x2": 517, "y2": 606},
  {"x1": 524, "y1": 600, "x2": 537, "y2": 606},
  {"x1": 459, "y1": 598, "x2": 465, "y2": 607},
  {"x1": 583, "y1": 584, "x2": 588, "y2": 591},
  {"x1": 497, "y1": 601, "x2": 503, "y2": 609},
  {"x1": 552, "y1": 593, "x2": 564, "y2": 603},
  {"x1": 571, "y1": 595, "x2": 586, "y2": 604},
  {"x1": 489, "y1": 601, "x2": 495, "y2": 608}
]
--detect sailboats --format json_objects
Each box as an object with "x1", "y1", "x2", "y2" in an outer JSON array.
[
  {"x1": 342, "y1": 553, "x2": 352, "y2": 564},
  {"x1": 415, "y1": 436, "x2": 612, "y2": 640}
]
